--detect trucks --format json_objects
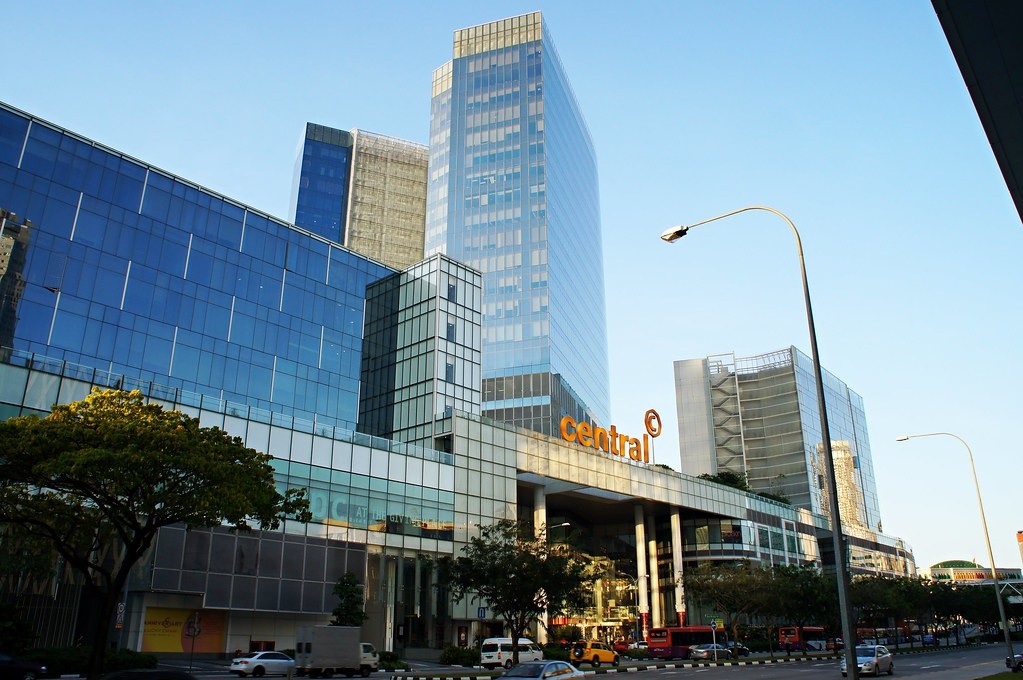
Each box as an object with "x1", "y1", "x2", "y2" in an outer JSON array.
[{"x1": 295, "y1": 625, "x2": 380, "y2": 678}]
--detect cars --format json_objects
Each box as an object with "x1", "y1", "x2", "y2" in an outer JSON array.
[
  {"x1": 691, "y1": 644, "x2": 732, "y2": 661},
  {"x1": 1006, "y1": 652, "x2": 1023, "y2": 672},
  {"x1": 840, "y1": 644, "x2": 894, "y2": 677},
  {"x1": 494, "y1": 660, "x2": 584, "y2": 680},
  {"x1": 628, "y1": 641, "x2": 648, "y2": 649},
  {"x1": 614, "y1": 640, "x2": 630, "y2": 653},
  {"x1": 923, "y1": 635, "x2": 940, "y2": 646},
  {"x1": 230, "y1": 651, "x2": 296, "y2": 678},
  {"x1": 718, "y1": 641, "x2": 749, "y2": 657}
]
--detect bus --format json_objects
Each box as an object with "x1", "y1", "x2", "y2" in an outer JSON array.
[
  {"x1": 856, "y1": 628, "x2": 887, "y2": 646},
  {"x1": 888, "y1": 628, "x2": 921, "y2": 644},
  {"x1": 779, "y1": 627, "x2": 827, "y2": 651},
  {"x1": 646, "y1": 625, "x2": 728, "y2": 660}
]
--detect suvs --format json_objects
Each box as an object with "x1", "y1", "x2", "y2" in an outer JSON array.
[
  {"x1": 570, "y1": 640, "x2": 621, "y2": 667},
  {"x1": 825, "y1": 638, "x2": 844, "y2": 651}
]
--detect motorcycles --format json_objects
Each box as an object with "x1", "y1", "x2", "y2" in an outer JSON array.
[{"x1": 560, "y1": 642, "x2": 568, "y2": 651}]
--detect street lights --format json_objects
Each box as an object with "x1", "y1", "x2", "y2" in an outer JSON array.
[
  {"x1": 896, "y1": 432, "x2": 1017, "y2": 670},
  {"x1": 660, "y1": 207, "x2": 859, "y2": 680},
  {"x1": 619, "y1": 572, "x2": 649, "y2": 650}
]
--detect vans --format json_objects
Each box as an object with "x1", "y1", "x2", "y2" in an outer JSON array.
[{"x1": 481, "y1": 638, "x2": 544, "y2": 670}]
